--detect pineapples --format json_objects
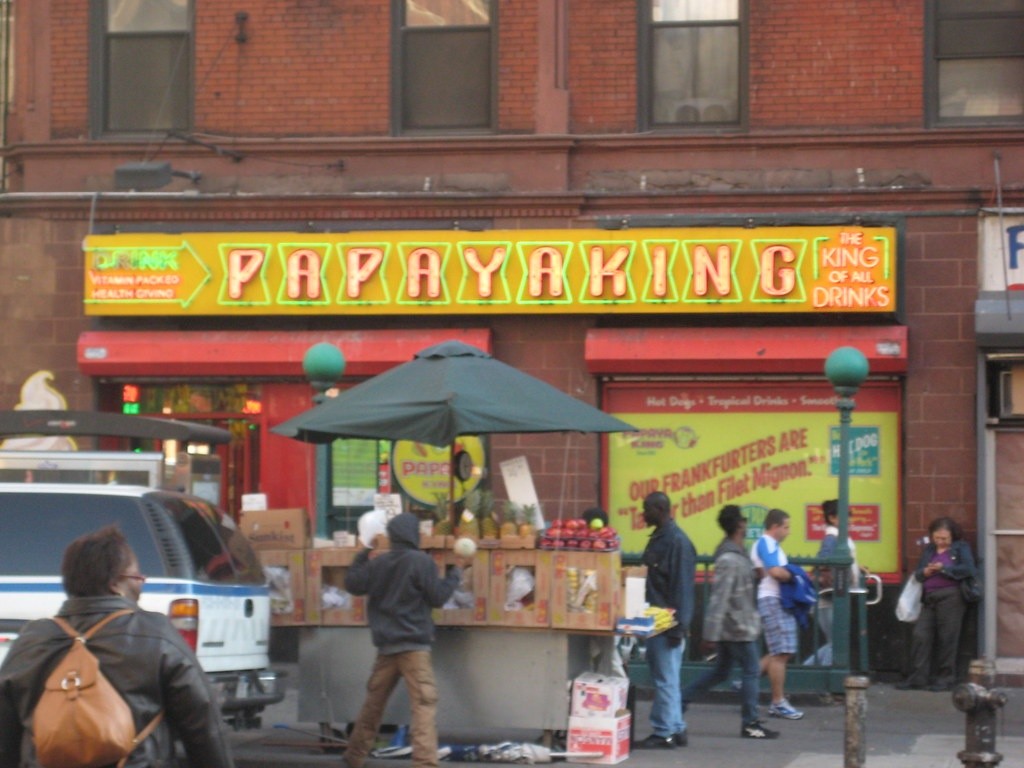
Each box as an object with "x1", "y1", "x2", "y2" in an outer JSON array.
[{"x1": 433, "y1": 490, "x2": 535, "y2": 539}]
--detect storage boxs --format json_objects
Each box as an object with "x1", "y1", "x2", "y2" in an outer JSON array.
[
  {"x1": 240, "y1": 506, "x2": 622, "y2": 630},
  {"x1": 564, "y1": 670, "x2": 632, "y2": 764}
]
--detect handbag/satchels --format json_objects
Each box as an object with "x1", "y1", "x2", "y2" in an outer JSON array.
[
  {"x1": 896, "y1": 572, "x2": 923, "y2": 623},
  {"x1": 810, "y1": 565, "x2": 834, "y2": 600},
  {"x1": 956, "y1": 542, "x2": 982, "y2": 603}
]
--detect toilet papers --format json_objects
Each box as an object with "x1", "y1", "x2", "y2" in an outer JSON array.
[{"x1": 625, "y1": 577, "x2": 647, "y2": 619}]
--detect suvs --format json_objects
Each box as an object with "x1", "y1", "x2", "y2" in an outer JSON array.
[{"x1": 0, "y1": 411, "x2": 287, "y2": 732}]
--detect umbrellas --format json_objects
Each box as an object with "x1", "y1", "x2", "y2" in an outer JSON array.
[{"x1": 270, "y1": 338, "x2": 642, "y2": 532}]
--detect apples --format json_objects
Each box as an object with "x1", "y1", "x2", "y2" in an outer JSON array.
[{"x1": 540, "y1": 519, "x2": 618, "y2": 548}]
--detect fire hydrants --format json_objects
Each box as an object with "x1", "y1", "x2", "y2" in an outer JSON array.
[{"x1": 952, "y1": 657, "x2": 1009, "y2": 768}]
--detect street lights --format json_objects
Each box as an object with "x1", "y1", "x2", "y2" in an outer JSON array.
[
  {"x1": 825, "y1": 346, "x2": 869, "y2": 558},
  {"x1": 304, "y1": 341, "x2": 345, "y2": 540}
]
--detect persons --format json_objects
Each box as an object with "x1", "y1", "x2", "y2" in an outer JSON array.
[
  {"x1": 338, "y1": 512, "x2": 476, "y2": 768},
  {"x1": 733, "y1": 509, "x2": 804, "y2": 719},
  {"x1": 895, "y1": 515, "x2": 978, "y2": 691},
  {"x1": 804, "y1": 500, "x2": 869, "y2": 664},
  {"x1": 0, "y1": 524, "x2": 235, "y2": 768},
  {"x1": 633, "y1": 491, "x2": 697, "y2": 750},
  {"x1": 681, "y1": 504, "x2": 785, "y2": 738}
]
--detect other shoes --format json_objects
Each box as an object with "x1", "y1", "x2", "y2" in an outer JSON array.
[
  {"x1": 742, "y1": 721, "x2": 779, "y2": 739},
  {"x1": 634, "y1": 731, "x2": 688, "y2": 749},
  {"x1": 768, "y1": 700, "x2": 805, "y2": 720},
  {"x1": 896, "y1": 676, "x2": 933, "y2": 691},
  {"x1": 924, "y1": 683, "x2": 956, "y2": 691}
]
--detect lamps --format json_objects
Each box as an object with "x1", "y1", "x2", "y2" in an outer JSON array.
[{"x1": 113, "y1": 160, "x2": 203, "y2": 192}]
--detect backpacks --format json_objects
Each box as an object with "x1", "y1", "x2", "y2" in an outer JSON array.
[{"x1": 32, "y1": 609, "x2": 165, "y2": 768}]
